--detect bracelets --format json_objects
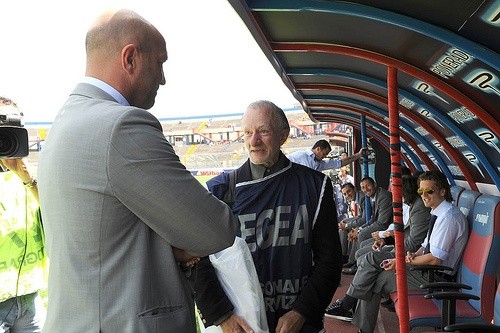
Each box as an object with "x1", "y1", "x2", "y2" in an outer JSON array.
[
  {"x1": 172, "y1": 255, "x2": 201, "y2": 269},
  {"x1": 21, "y1": 175, "x2": 38, "y2": 189}
]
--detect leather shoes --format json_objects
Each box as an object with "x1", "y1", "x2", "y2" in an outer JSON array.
[
  {"x1": 343, "y1": 262, "x2": 354, "y2": 268},
  {"x1": 342, "y1": 266, "x2": 357, "y2": 275}
]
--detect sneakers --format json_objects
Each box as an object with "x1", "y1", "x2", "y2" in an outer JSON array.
[{"x1": 323, "y1": 298, "x2": 353, "y2": 322}]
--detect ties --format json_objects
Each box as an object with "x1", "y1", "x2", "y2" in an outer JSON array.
[
  {"x1": 372, "y1": 201, "x2": 375, "y2": 218},
  {"x1": 425, "y1": 215, "x2": 437, "y2": 254},
  {"x1": 354, "y1": 204, "x2": 357, "y2": 216}
]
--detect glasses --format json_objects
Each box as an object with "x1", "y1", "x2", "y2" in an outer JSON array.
[{"x1": 417, "y1": 187, "x2": 436, "y2": 196}]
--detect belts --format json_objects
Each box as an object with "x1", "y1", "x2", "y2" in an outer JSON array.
[{"x1": 434, "y1": 270, "x2": 456, "y2": 281}]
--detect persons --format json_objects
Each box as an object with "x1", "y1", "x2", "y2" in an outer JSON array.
[
  {"x1": 288, "y1": 138, "x2": 369, "y2": 173},
  {"x1": 335, "y1": 180, "x2": 366, "y2": 264},
  {"x1": 324, "y1": 170, "x2": 469, "y2": 333},
  {"x1": 342, "y1": 176, "x2": 393, "y2": 274},
  {"x1": 330, "y1": 150, "x2": 354, "y2": 225},
  {"x1": 0, "y1": 95, "x2": 46, "y2": 333},
  {"x1": 35, "y1": 9, "x2": 237, "y2": 333},
  {"x1": 194, "y1": 124, "x2": 353, "y2": 148},
  {"x1": 354, "y1": 194, "x2": 411, "y2": 248},
  {"x1": 189, "y1": 100, "x2": 342, "y2": 333},
  {"x1": 355, "y1": 175, "x2": 432, "y2": 312}
]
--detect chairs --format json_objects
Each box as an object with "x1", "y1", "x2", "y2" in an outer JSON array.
[{"x1": 391, "y1": 185, "x2": 500, "y2": 333}]
